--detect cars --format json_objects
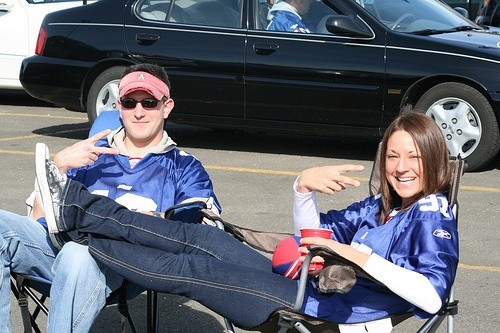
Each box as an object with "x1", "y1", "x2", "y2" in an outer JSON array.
[
  {"x1": 18, "y1": 0, "x2": 500, "y2": 173},
  {"x1": 0, "y1": 0, "x2": 96, "y2": 101}
]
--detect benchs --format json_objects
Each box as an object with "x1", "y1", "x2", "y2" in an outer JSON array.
[{"x1": 143, "y1": 0, "x2": 240, "y2": 25}]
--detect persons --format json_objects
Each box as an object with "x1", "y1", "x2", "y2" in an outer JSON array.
[
  {"x1": 266, "y1": 0, "x2": 313, "y2": 33},
  {"x1": 35, "y1": 111, "x2": 458, "y2": 330},
  {"x1": 0, "y1": 65, "x2": 222, "y2": 333}
]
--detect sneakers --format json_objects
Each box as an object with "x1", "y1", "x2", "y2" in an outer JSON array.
[{"x1": 35, "y1": 142, "x2": 70, "y2": 249}]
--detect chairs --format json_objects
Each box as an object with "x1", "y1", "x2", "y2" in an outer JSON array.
[
  {"x1": 10, "y1": 108, "x2": 208, "y2": 333},
  {"x1": 199, "y1": 154, "x2": 463, "y2": 333}
]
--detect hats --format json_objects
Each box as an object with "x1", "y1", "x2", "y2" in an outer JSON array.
[{"x1": 118, "y1": 71, "x2": 170, "y2": 100}]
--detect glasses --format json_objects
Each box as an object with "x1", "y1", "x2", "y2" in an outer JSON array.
[{"x1": 120, "y1": 98, "x2": 160, "y2": 109}]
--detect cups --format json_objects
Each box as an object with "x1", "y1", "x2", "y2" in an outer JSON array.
[{"x1": 299, "y1": 228, "x2": 333, "y2": 271}]
